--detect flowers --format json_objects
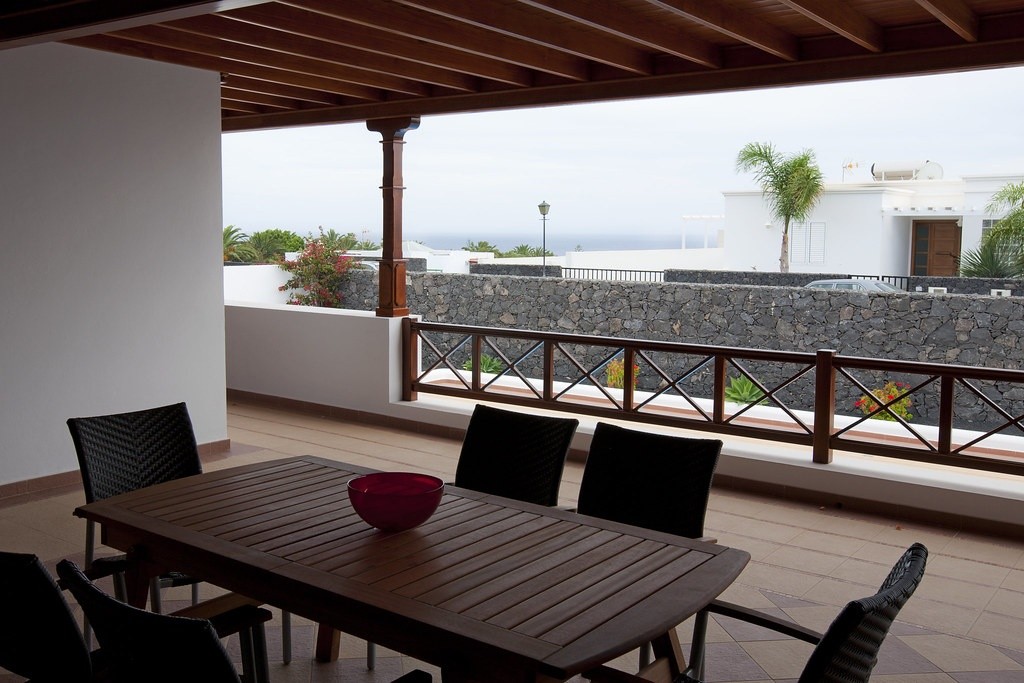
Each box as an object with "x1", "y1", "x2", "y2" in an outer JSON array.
[{"x1": 855, "y1": 379, "x2": 915, "y2": 423}]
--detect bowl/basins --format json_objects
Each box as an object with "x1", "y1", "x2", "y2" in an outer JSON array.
[{"x1": 347, "y1": 472, "x2": 444, "y2": 531}]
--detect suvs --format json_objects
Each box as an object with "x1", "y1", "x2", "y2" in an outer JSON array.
[{"x1": 804, "y1": 279, "x2": 911, "y2": 295}]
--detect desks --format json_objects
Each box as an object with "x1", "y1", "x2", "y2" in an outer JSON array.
[{"x1": 69, "y1": 451, "x2": 754, "y2": 683}]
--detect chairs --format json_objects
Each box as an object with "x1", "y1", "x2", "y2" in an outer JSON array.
[
  {"x1": 0, "y1": 548, "x2": 434, "y2": 683},
  {"x1": 575, "y1": 420, "x2": 724, "y2": 672},
  {"x1": 366, "y1": 404, "x2": 579, "y2": 672},
  {"x1": 584, "y1": 541, "x2": 931, "y2": 683},
  {"x1": 66, "y1": 402, "x2": 294, "y2": 667}
]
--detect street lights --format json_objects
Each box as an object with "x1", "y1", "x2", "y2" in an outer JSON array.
[{"x1": 538, "y1": 200, "x2": 550, "y2": 276}]
